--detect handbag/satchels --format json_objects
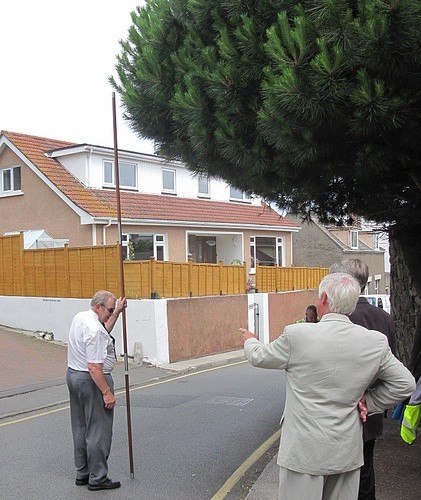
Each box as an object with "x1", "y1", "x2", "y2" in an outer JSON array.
[{"x1": 391, "y1": 403, "x2": 404, "y2": 423}]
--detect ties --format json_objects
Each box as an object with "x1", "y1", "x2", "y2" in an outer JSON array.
[{"x1": 102, "y1": 323, "x2": 118, "y2": 362}]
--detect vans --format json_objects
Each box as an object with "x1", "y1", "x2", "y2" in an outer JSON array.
[{"x1": 360, "y1": 294, "x2": 390, "y2": 314}]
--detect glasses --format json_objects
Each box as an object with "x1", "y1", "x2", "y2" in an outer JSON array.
[{"x1": 100, "y1": 304, "x2": 114, "y2": 314}]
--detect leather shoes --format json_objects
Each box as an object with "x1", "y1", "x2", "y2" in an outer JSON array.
[
  {"x1": 87, "y1": 479, "x2": 121, "y2": 492},
  {"x1": 75, "y1": 478, "x2": 90, "y2": 486}
]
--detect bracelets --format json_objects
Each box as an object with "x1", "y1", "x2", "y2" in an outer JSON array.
[{"x1": 102, "y1": 386, "x2": 110, "y2": 394}]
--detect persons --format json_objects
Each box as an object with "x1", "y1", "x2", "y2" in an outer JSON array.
[
  {"x1": 238, "y1": 272, "x2": 417, "y2": 500},
  {"x1": 295, "y1": 305, "x2": 320, "y2": 323},
  {"x1": 328, "y1": 258, "x2": 397, "y2": 500},
  {"x1": 65, "y1": 289, "x2": 128, "y2": 491}
]
